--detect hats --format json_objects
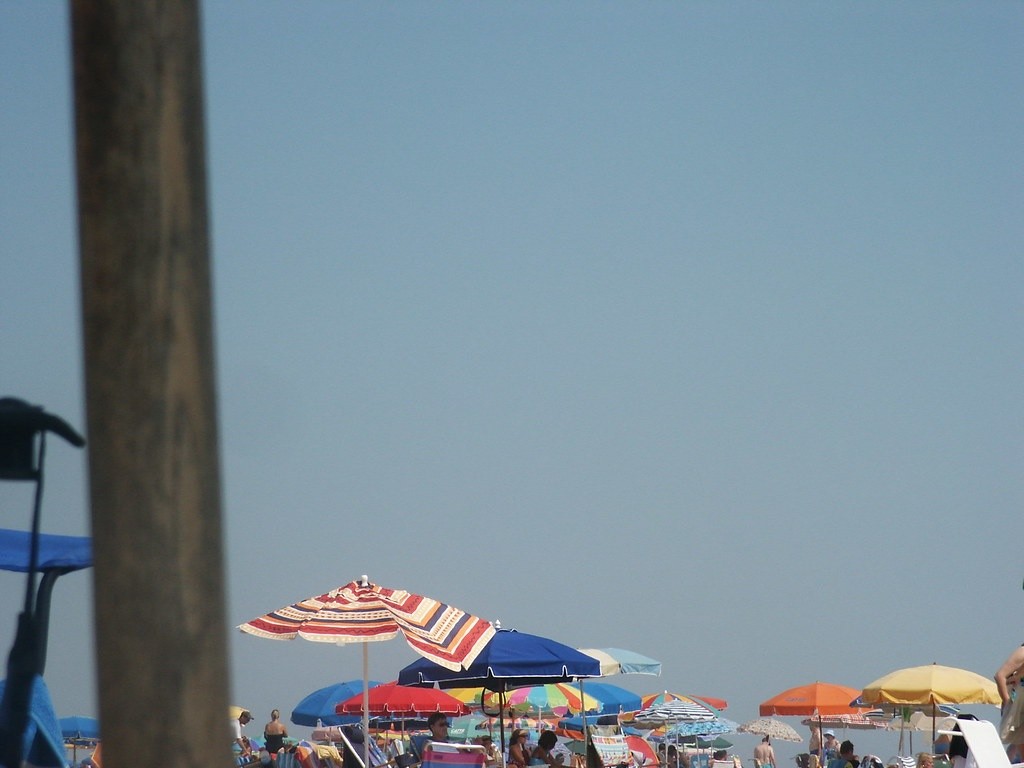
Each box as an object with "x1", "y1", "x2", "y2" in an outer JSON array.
[
  {"x1": 823, "y1": 730, "x2": 835, "y2": 737},
  {"x1": 242, "y1": 711, "x2": 255, "y2": 720}
]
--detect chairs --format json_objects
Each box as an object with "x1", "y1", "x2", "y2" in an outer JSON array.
[{"x1": 235, "y1": 718, "x2": 1008, "y2": 768}]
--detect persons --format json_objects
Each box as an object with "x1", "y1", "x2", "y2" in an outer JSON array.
[
  {"x1": 916, "y1": 735, "x2": 950, "y2": 768},
  {"x1": 838, "y1": 740, "x2": 860, "y2": 768},
  {"x1": 754, "y1": 738, "x2": 777, "y2": 768},
  {"x1": 668, "y1": 745, "x2": 689, "y2": 768},
  {"x1": 823, "y1": 730, "x2": 841, "y2": 760},
  {"x1": 264, "y1": 710, "x2": 288, "y2": 754},
  {"x1": 659, "y1": 743, "x2": 665, "y2": 755},
  {"x1": 428, "y1": 713, "x2": 459, "y2": 744},
  {"x1": 232, "y1": 711, "x2": 254, "y2": 756},
  {"x1": 532, "y1": 731, "x2": 562, "y2": 768},
  {"x1": 994, "y1": 644, "x2": 1024, "y2": 764},
  {"x1": 809, "y1": 725, "x2": 824, "y2": 757},
  {"x1": 507, "y1": 729, "x2": 528, "y2": 768}
]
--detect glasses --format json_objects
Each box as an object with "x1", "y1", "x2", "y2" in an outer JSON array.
[
  {"x1": 519, "y1": 735, "x2": 528, "y2": 738},
  {"x1": 438, "y1": 721, "x2": 449, "y2": 727}
]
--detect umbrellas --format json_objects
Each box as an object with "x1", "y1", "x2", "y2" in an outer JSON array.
[
  {"x1": 236, "y1": 574, "x2": 741, "y2": 768},
  {"x1": 55, "y1": 716, "x2": 102, "y2": 768},
  {"x1": 737, "y1": 680, "x2": 888, "y2": 768},
  {"x1": 228, "y1": 706, "x2": 249, "y2": 720},
  {"x1": 848, "y1": 662, "x2": 1016, "y2": 757}
]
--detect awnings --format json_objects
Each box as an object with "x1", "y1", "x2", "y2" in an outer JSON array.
[{"x1": 0, "y1": 529, "x2": 94, "y2": 768}]
